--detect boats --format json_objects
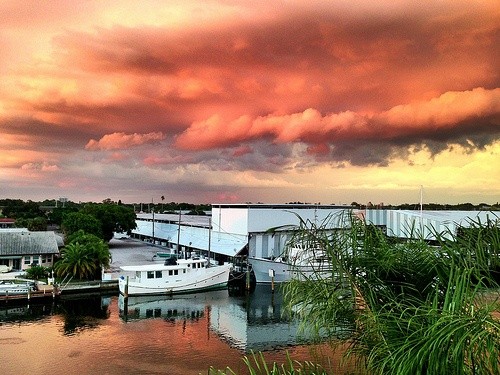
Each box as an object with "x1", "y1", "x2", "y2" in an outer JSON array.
[
  {"x1": 245, "y1": 209, "x2": 367, "y2": 283},
  {"x1": 118, "y1": 285, "x2": 228, "y2": 313},
  {"x1": 0, "y1": 278, "x2": 62, "y2": 300},
  {"x1": 118, "y1": 256, "x2": 232, "y2": 296}
]
